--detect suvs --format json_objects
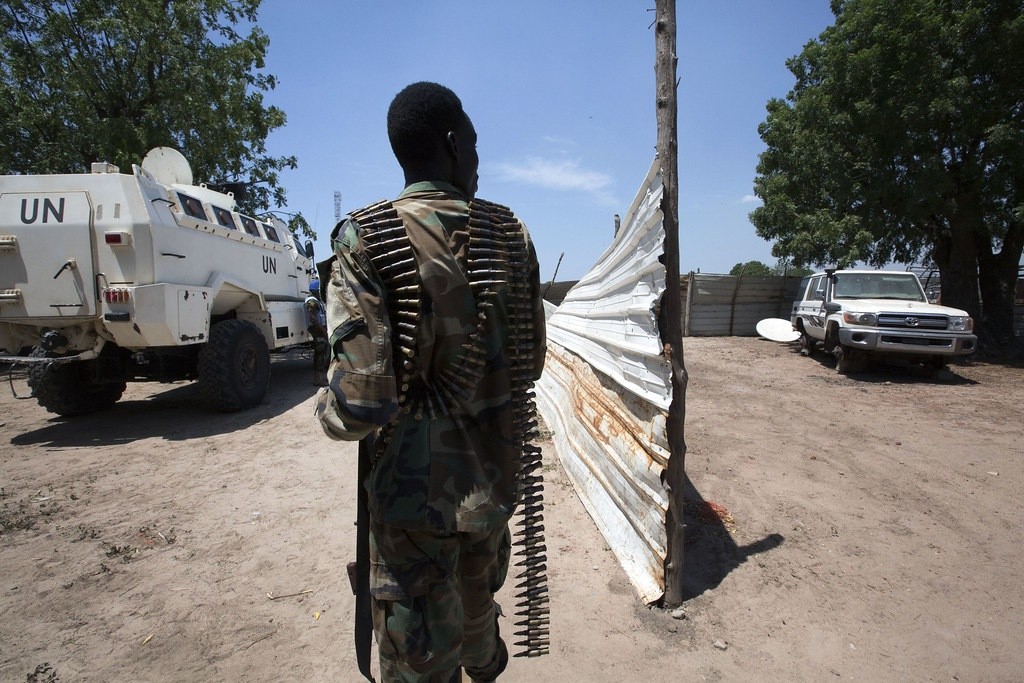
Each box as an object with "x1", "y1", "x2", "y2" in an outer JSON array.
[{"x1": 789, "y1": 268, "x2": 977, "y2": 369}]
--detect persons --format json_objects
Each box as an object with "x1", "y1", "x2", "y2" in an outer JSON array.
[
  {"x1": 316, "y1": 83, "x2": 546, "y2": 683},
  {"x1": 304, "y1": 282, "x2": 330, "y2": 386}
]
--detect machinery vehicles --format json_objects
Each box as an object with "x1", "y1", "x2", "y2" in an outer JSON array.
[{"x1": 0, "y1": 146, "x2": 320, "y2": 417}]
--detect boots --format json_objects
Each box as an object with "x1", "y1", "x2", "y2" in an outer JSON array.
[{"x1": 315, "y1": 366, "x2": 329, "y2": 386}]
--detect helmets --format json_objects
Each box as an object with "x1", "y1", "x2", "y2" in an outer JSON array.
[{"x1": 309, "y1": 280, "x2": 319, "y2": 292}]
